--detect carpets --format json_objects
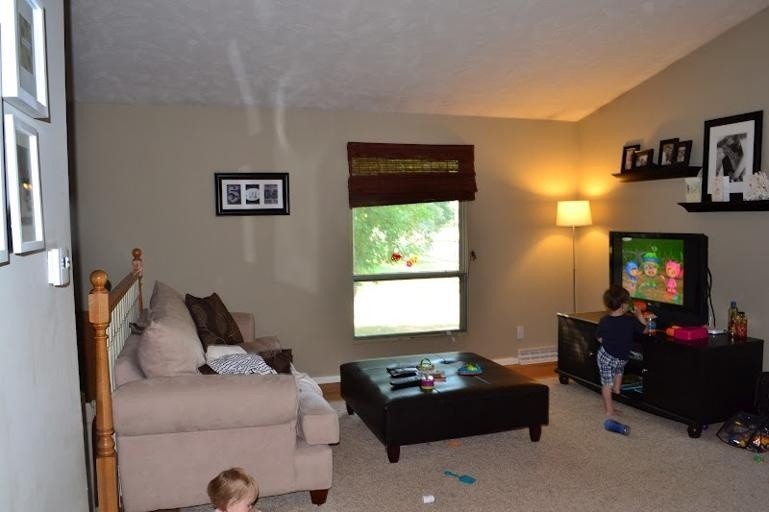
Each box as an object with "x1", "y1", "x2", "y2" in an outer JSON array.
[{"x1": 176, "y1": 373, "x2": 747, "y2": 510}]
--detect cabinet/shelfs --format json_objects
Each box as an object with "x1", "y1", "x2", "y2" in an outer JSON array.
[{"x1": 556, "y1": 311, "x2": 767, "y2": 443}]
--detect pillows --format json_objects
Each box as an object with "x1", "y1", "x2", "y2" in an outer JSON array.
[{"x1": 138, "y1": 279, "x2": 297, "y2": 376}]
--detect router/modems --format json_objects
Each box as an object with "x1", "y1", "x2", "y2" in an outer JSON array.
[{"x1": 708, "y1": 316, "x2": 724, "y2": 334}]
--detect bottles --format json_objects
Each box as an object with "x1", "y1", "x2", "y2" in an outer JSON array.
[
  {"x1": 647, "y1": 317, "x2": 656, "y2": 331},
  {"x1": 604, "y1": 418, "x2": 630, "y2": 435},
  {"x1": 727, "y1": 301, "x2": 738, "y2": 335},
  {"x1": 736, "y1": 313, "x2": 747, "y2": 336}
]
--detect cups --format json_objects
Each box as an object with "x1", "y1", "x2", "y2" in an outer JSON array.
[
  {"x1": 416, "y1": 357, "x2": 435, "y2": 390},
  {"x1": 712, "y1": 177, "x2": 730, "y2": 202},
  {"x1": 743, "y1": 175, "x2": 758, "y2": 201},
  {"x1": 685, "y1": 177, "x2": 703, "y2": 204}
]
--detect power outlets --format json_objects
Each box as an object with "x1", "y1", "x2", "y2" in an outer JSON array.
[{"x1": 515, "y1": 323, "x2": 526, "y2": 341}]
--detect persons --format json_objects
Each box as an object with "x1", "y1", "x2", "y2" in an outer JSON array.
[
  {"x1": 624, "y1": 144, "x2": 686, "y2": 169},
  {"x1": 716, "y1": 135, "x2": 734, "y2": 183},
  {"x1": 207, "y1": 466, "x2": 261, "y2": 512},
  {"x1": 595, "y1": 284, "x2": 647, "y2": 415},
  {"x1": 726, "y1": 133, "x2": 746, "y2": 182}
]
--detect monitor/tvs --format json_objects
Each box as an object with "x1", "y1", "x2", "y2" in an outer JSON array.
[{"x1": 609, "y1": 231, "x2": 708, "y2": 332}]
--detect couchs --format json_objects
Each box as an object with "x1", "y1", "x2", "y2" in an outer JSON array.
[
  {"x1": 111, "y1": 306, "x2": 341, "y2": 511},
  {"x1": 340, "y1": 348, "x2": 550, "y2": 462}
]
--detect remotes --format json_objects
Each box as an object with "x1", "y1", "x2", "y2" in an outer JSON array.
[
  {"x1": 390, "y1": 367, "x2": 416, "y2": 376},
  {"x1": 386, "y1": 365, "x2": 415, "y2": 371},
  {"x1": 390, "y1": 375, "x2": 422, "y2": 386}
]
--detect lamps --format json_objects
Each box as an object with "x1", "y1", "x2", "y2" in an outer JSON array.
[{"x1": 553, "y1": 198, "x2": 593, "y2": 314}]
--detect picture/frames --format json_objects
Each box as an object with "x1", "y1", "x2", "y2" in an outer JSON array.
[
  {"x1": 2, "y1": 112, "x2": 47, "y2": 257},
  {"x1": 1, "y1": 1, "x2": 51, "y2": 122},
  {"x1": 700, "y1": 108, "x2": 766, "y2": 204},
  {"x1": 618, "y1": 136, "x2": 694, "y2": 172},
  {"x1": 212, "y1": 170, "x2": 291, "y2": 216}
]
